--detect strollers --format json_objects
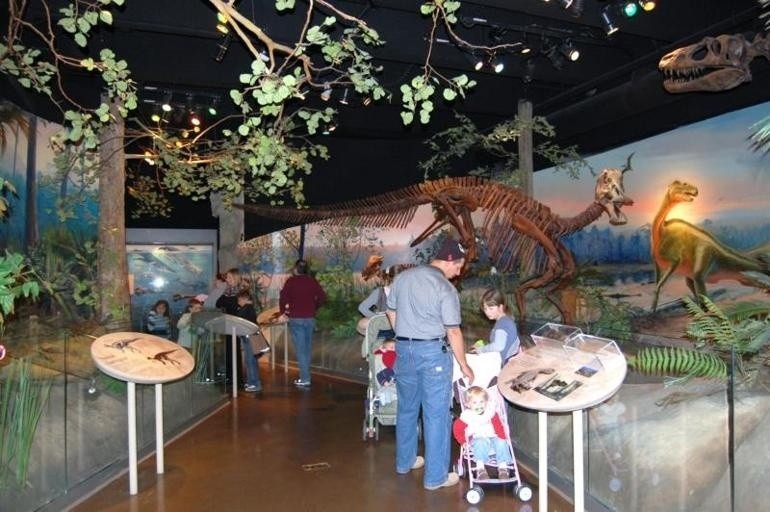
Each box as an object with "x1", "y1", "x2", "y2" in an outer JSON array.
[
  {"x1": 360, "y1": 312, "x2": 423, "y2": 442},
  {"x1": 452, "y1": 350, "x2": 534, "y2": 506}
]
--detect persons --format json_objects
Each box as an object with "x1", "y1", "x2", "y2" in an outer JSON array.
[
  {"x1": 215, "y1": 268, "x2": 247, "y2": 391},
  {"x1": 234, "y1": 290, "x2": 262, "y2": 391},
  {"x1": 359, "y1": 264, "x2": 406, "y2": 317},
  {"x1": 378, "y1": 338, "x2": 396, "y2": 386},
  {"x1": 470, "y1": 288, "x2": 522, "y2": 369},
  {"x1": 386, "y1": 242, "x2": 475, "y2": 490},
  {"x1": 147, "y1": 300, "x2": 171, "y2": 340},
  {"x1": 453, "y1": 386, "x2": 510, "y2": 479},
  {"x1": 175, "y1": 298, "x2": 202, "y2": 356},
  {"x1": 279, "y1": 259, "x2": 326, "y2": 385},
  {"x1": 204, "y1": 273, "x2": 226, "y2": 379}
]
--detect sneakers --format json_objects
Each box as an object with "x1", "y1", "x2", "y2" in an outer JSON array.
[
  {"x1": 243, "y1": 383, "x2": 262, "y2": 392},
  {"x1": 292, "y1": 378, "x2": 311, "y2": 386},
  {"x1": 395, "y1": 455, "x2": 425, "y2": 475},
  {"x1": 475, "y1": 469, "x2": 489, "y2": 480},
  {"x1": 423, "y1": 472, "x2": 461, "y2": 491},
  {"x1": 497, "y1": 468, "x2": 509, "y2": 480}
]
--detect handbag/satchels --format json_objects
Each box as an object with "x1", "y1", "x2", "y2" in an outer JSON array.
[
  {"x1": 247, "y1": 331, "x2": 271, "y2": 358},
  {"x1": 356, "y1": 317, "x2": 370, "y2": 338}
]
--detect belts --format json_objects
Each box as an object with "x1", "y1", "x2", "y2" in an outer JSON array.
[{"x1": 396, "y1": 336, "x2": 447, "y2": 342}]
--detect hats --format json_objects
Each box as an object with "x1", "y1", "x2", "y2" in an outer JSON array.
[{"x1": 434, "y1": 239, "x2": 466, "y2": 261}]
